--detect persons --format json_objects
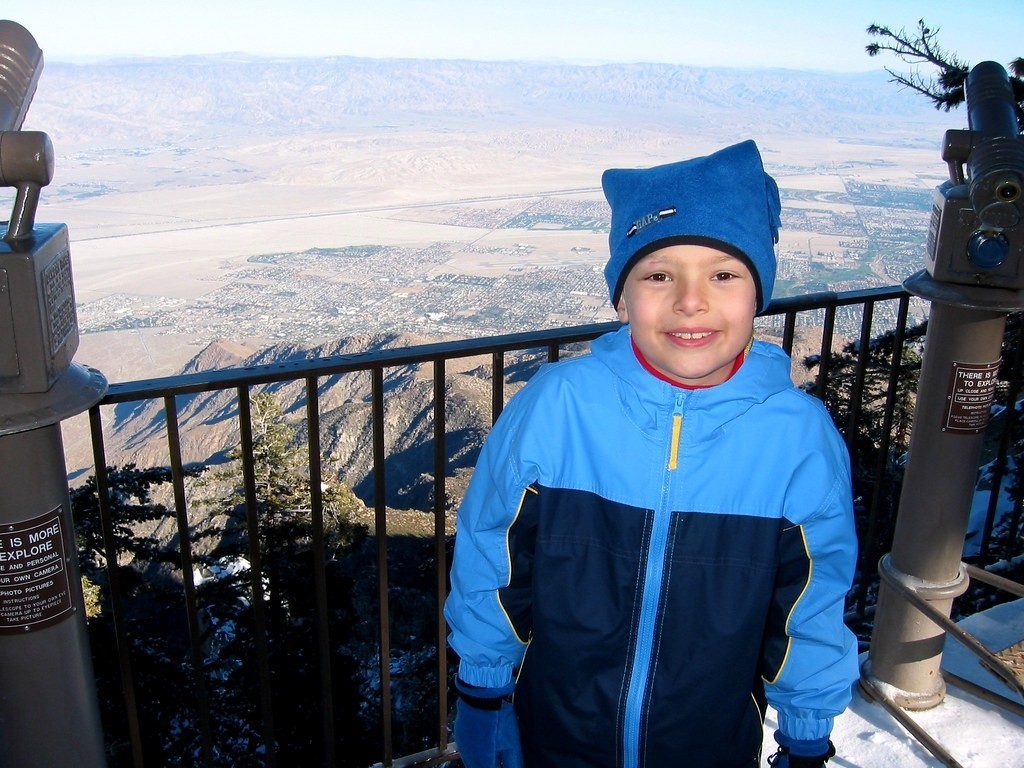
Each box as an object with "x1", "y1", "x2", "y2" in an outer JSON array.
[{"x1": 444, "y1": 134, "x2": 860, "y2": 768}]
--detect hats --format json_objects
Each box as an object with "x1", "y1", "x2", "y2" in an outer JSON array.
[{"x1": 601, "y1": 139, "x2": 783, "y2": 318}]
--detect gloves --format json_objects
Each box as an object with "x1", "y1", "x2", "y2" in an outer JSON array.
[
  {"x1": 768, "y1": 729, "x2": 835, "y2": 768},
  {"x1": 449, "y1": 673, "x2": 522, "y2": 768}
]
